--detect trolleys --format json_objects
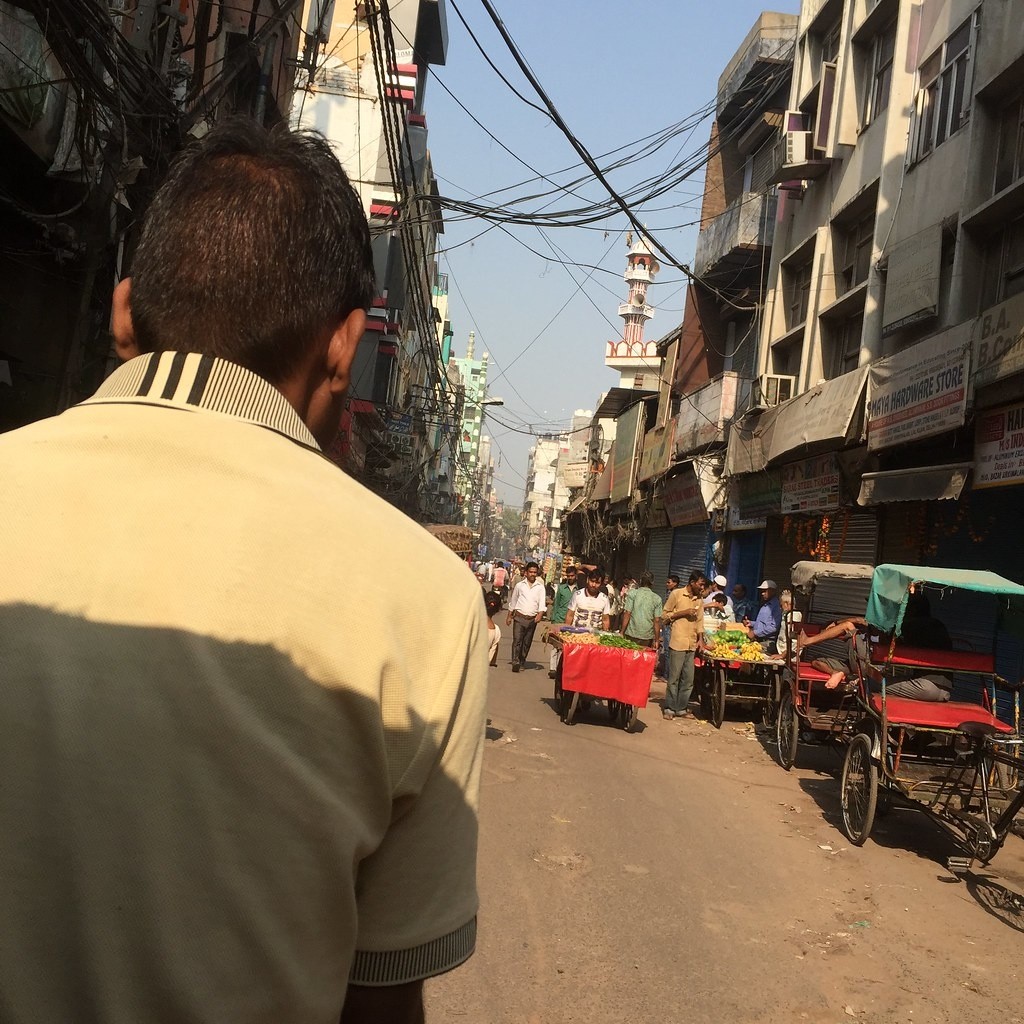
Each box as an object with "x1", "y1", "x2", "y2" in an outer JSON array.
[
  {"x1": 696, "y1": 646, "x2": 789, "y2": 729},
  {"x1": 541, "y1": 627, "x2": 657, "y2": 733}
]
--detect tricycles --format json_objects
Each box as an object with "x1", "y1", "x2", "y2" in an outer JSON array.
[
  {"x1": 776, "y1": 558, "x2": 882, "y2": 770},
  {"x1": 836, "y1": 562, "x2": 1023, "y2": 872}
]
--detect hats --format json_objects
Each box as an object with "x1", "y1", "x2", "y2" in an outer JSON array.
[
  {"x1": 713, "y1": 575, "x2": 727, "y2": 587},
  {"x1": 756, "y1": 579, "x2": 777, "y2": 590}
]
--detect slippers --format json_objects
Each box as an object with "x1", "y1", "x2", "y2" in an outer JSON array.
[
  {"x1": 663, "y1": 711, "x2": 674, "y2": 720},
  {"x1": 679, "y1": 712, "x2": 697, "y2": 720}
]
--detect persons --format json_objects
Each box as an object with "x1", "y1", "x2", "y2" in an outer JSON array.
[
  {"x1": 577, "y1": 568, "x2": 640, "y2": 633},
  {"x1": 769, "y1": 590, "x2": 804, "y2": 664},
  {"x1": 505, "y1": 561, "x2": 546, "y2": 672},
  {"x1": 661, "y1": 570, "x2": 706, "y2": 721},
  {"x1": 874, "y1": 591, "x2": 955, "y2": 702},
  {"x1": 564, "y1": 569, "x2": 610, "y2": 714},
  {"x1": 799, "y1": 616, "x2": 872, "y2": 690},
  {"x1": 743, "y1": 580, "x2": 782, "y2": 686},
  {"x1": 471, "y1": 557, "x2": 558, "y2": 621},
  {"x1": 700, "y1": 575, "x2": 759, "y2": 625},
  {"x1": 619, "y1": 570, "x2": 662, "y2": 651},
  {"x1": 485, "y1": 590, "x2": 502, "y2": 666},
  {"x1": 653, "y1": 575, "x2": 681, "y2": 684},
  {"x1": 547, "y1": 565, "x2": 581, "y2": 680},
  {"x1": 0, "y1": 118, "x2": 489, "y2": 1024}
]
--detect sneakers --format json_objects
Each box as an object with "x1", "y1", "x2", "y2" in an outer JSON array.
[
  {"x1": 548, "y1": 669, "x2": 557, "y2": 679},
  {"x1": 512, "y1": 664, "x2": 524, "y2": 672}
]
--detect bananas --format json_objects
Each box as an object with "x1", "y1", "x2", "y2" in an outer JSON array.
[{"x1": 710, "y1": 640, "x2": 764, "y2": 661}]
